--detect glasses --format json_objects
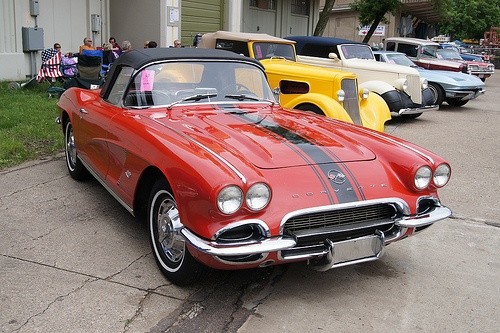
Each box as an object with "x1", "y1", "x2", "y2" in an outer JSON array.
[
  {"x1": 56, "y1": 47, "x2": 61, "y2": 48},
  {"x1": 178, "y1": 44, "x2": 181, "y2": 45},
  {"x1": 89, "y1": 41, "x2": 92, "y2": 42},
  {"x1": 110, "y1": 42, "x2": 113, "y2": 43}
]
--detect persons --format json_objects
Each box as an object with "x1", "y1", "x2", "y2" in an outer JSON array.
[
  {"x1": 173, "y1": 40, "x2": 183, "y2": 48},
  {"x1": 53, "y1": 43, "x2": 61, "y2": 51},
  {"x1": 102, "y1": 37, "x2": 120, "y2": 50},
  {"x1": 80, "y1": 38, "x2": 96, "y2": 54},
  {"x1": 379, "y1": 38, "x2": 386, "y2": 51},
  {"x1": 148, "y1": 41, "x2": 157, "y2": 48},
  {"x1": 121, "y1": 41, "x2": 131, "y2": 51},
  {"x1": 264, "y1": 42, "x2": 277, "y2": 58}
]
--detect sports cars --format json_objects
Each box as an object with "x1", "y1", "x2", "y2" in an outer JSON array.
[
  {"x1": 369, "y1": 50, "x2": 486, "y2": 107},
  {"x1": 55, "y1": 49, "x2": 453, "y2": 286}
]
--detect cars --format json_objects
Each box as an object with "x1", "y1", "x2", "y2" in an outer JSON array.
[
  {"x1": 380, "y1": 37, "x2": 495, "y2": 82},
  {"x1": 153, "y1": 31, "x2": 392, "y2": 134},
  {"x1": 266, "y1": 36, "x2": 440, "y2": 118},
  {"x1": 435, "y1": 37, "x2": 493, "y2": 66}
]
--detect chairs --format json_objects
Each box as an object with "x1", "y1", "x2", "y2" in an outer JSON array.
[
  {"x1": 125, "y1": 90, "x2": 170, "y2": 106},
  {"x1": 36, "y1": 48, "x2": 120, "y2": 99}
]
